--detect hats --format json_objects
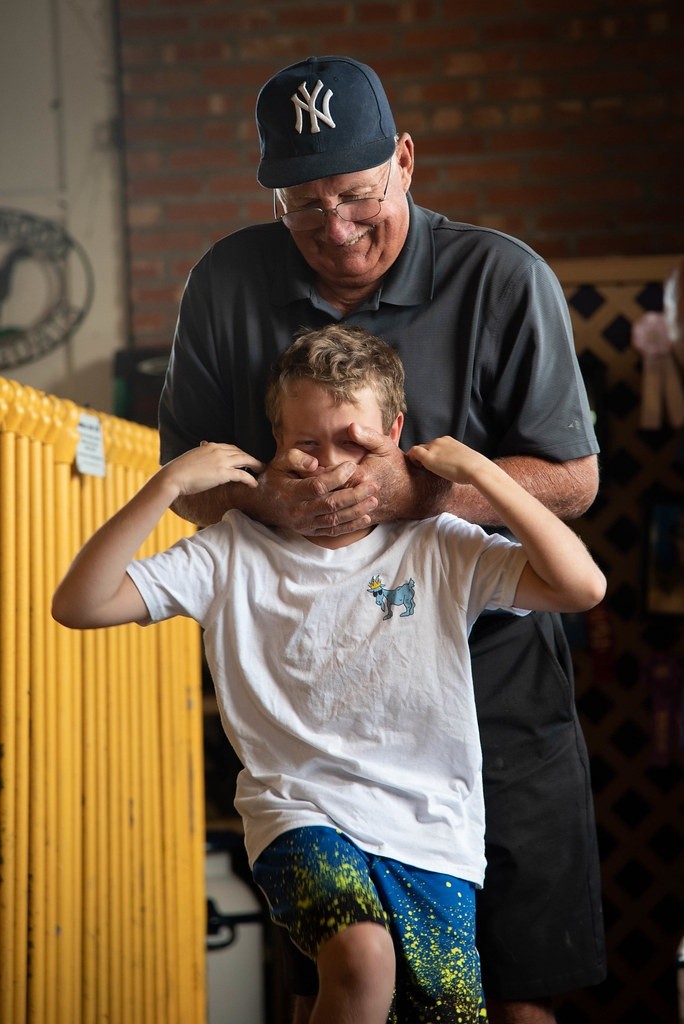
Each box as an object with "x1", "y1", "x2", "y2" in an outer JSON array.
[{"x1": 254, "y1": 56, "x2": 396, "y2": 188}]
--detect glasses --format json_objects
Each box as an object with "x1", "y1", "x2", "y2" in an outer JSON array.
[{"x1": 272, "y1": 161, "x2": 392, "y2": 231}]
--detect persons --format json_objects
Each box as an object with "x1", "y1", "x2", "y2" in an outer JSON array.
[
  {"x1": 158, "y1": 55, "x2": 616, "y2": 1024},
  {"x1": 51, "y1": 328, "x2": 607, "y2": 1024}
]
